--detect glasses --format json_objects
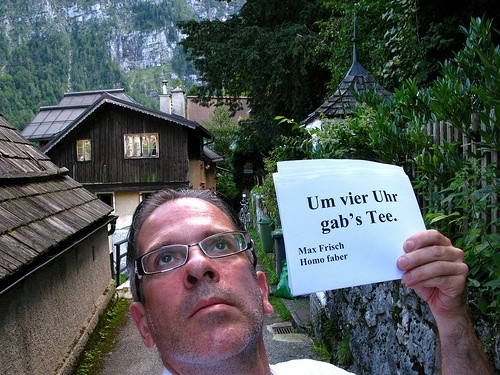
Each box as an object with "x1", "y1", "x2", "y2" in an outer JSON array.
[{"x1": 133, "y1": 230, "x2": 257, "y2": 299}]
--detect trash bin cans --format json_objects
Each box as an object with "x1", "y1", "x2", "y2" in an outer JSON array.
[
  {"x1": 257, "y1": 215, "x2": 277, "y2": 253},
  {"x1": 270, "y1": 229, "x2": 286, "y2": 282}
]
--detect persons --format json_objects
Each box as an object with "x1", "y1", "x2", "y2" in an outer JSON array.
[{"x1": 125, "y1": 186, "x2": 497, "y2": 373}]
